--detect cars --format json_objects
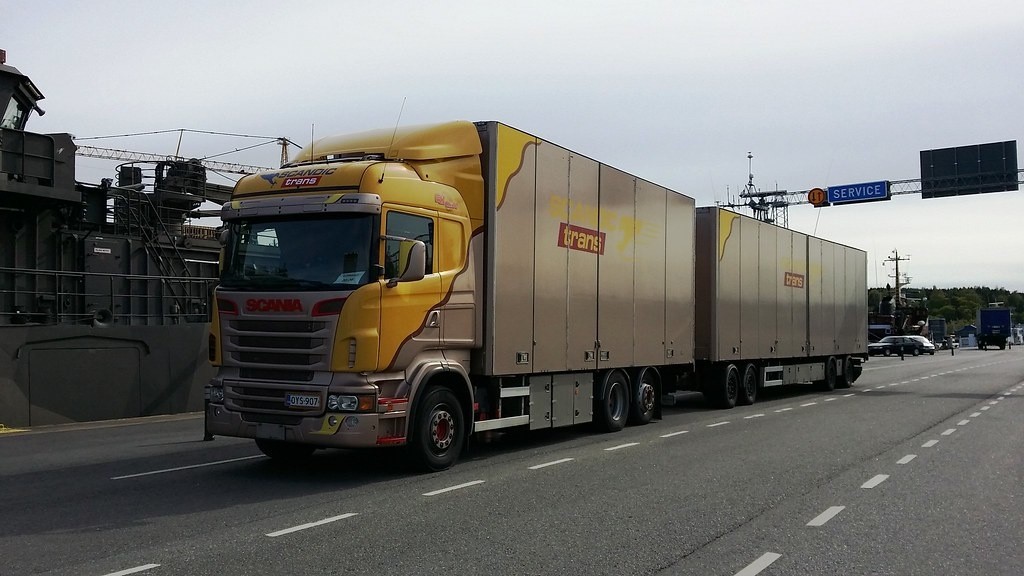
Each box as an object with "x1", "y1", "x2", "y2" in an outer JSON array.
[
  {"x1": 908, "y1": 336, "x2": 935, "y2": 356},
  {"x1": 868, "y1": 336, "x2": 919, "y2": 356}
]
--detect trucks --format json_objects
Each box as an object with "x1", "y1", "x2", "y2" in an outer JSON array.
[{"x1": 975, "y1": 308, "x2": 1011, "y2": 349}]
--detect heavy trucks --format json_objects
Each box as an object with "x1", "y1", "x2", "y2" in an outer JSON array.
[{"x1": 205, "y1": 120, "x2": 867, "y2": 472}]
